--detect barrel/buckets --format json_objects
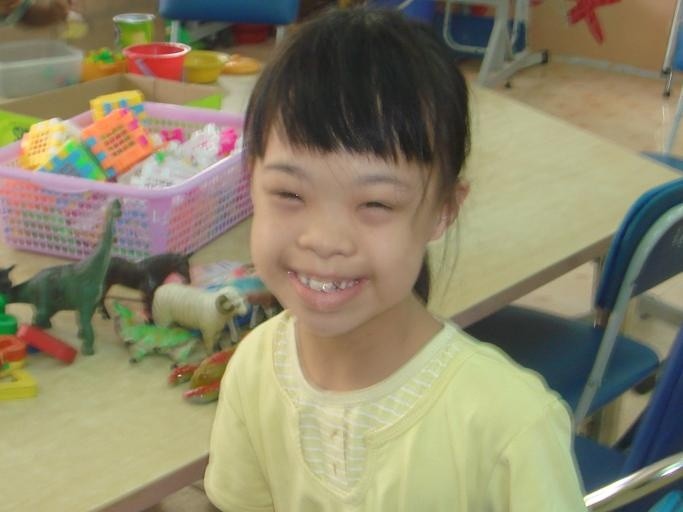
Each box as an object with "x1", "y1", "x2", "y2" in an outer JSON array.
[{"x1": 122, "y1": 41, "x2": 191, "y2": 81}]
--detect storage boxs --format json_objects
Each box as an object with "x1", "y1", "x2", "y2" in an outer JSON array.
[{"x1": 0, "y1": 73, "x2": 223, "y2": 146}]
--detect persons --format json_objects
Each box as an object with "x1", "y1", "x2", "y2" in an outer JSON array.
[{"x1": 201, "y1": 6, "x2": 590, "y2": 512}]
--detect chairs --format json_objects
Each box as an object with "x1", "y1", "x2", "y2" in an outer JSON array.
[
  {"x1": 461, "y1": 176, "x2": 683, "y2": 511},
  {"x1": 636, "y1": 18, "x2": 683, "y2": 154},
  {"x1": 372, "y1": 0, "x2": 550, "y2": 93},
  {"x1": 157, "y1": 0, "x2": 300, "y2": 63},
  {"x1": 586, "y1": 147, "x2": 683, "y2": 330}
]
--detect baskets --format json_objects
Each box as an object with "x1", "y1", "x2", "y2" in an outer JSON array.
[{"x1": 0, "y1": 101, "x2": 254, "y2": 261}]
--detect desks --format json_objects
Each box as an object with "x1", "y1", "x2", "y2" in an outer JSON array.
[{"x1": 0, "y1": 52, "x2": 683, "y2": 511}]
